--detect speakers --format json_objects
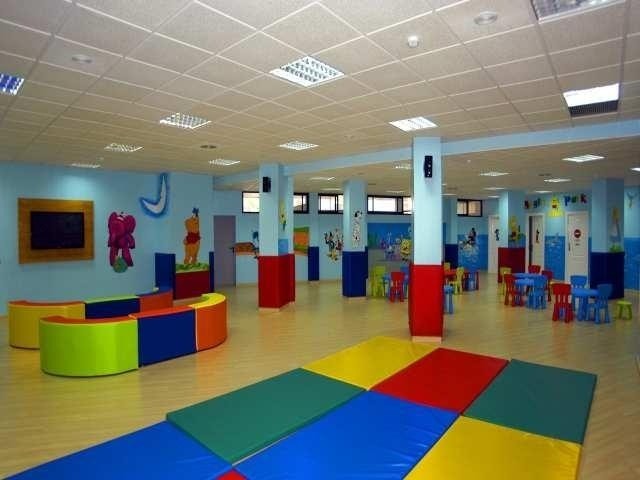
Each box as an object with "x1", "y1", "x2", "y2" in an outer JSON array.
[
  {"x1": 262, "y1": 177, "x2": 272, "y2": 192},
  {"x1": 425, "y1": 156, "x2": 432, "y2": 178}
]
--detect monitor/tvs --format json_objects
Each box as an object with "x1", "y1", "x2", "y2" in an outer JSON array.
[{"x1": 29, "y1": 209, "x2": 85, "y2": 250}]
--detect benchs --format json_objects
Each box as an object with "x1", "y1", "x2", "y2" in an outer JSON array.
[
  {"x1": 41, "y1": 291, "x2": 230, "y2": 380},
  {"x1": 7, "y1": 283, "x2": 176, "y2": 352}
]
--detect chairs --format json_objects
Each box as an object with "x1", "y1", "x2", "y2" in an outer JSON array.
[{"x1": 368, "y1": 259, "x2": 635, "y2": 329}]
